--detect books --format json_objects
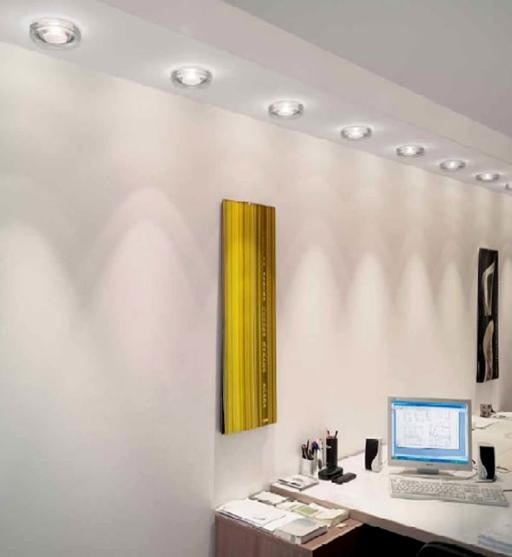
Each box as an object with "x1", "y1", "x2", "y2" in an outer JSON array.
[{"x1": 216, "y1": 487, "x2": 349, "y2": 544}]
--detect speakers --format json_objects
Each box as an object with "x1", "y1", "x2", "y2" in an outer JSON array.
[
  {"x1": 479, "y1": 443, "x2": 495, "y2": 479},
  {"x1": 365, "y1": 438, "x2": 382, "y2": 472}
]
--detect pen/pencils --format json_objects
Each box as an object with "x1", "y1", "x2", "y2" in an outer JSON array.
[
  {"x1": 312, "y1": 438, "x2": 323, "y2": 448},
  {"x1": 301, "y1": 439, "x2": 313, "y2": 460},
  {"x1": 327, "y1": 430, "x2": 338, "y2": 439}
]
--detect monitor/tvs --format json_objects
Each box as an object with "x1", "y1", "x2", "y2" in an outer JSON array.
[{"x1": 387, "y1": 396, "x2": 473, "y2": 479}]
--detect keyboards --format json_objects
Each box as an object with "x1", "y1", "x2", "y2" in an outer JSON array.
[{"x1": 389, "y1": 479, "x2": 509, "y2": 507}]
